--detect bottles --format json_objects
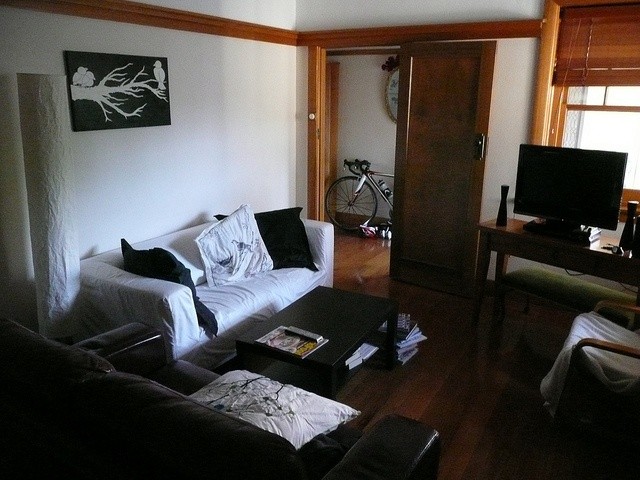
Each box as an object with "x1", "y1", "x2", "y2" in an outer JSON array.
[{"x1": 378, "y1": 179, "x2": 393, "y2": 198}]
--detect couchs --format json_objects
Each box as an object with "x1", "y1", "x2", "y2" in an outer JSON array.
[
  {"x1": 78, "y1": 217, "x2": 337, "y2": 361},
  {"x1": 0, "y1": 312, "x2": 443, "y2": 479}
]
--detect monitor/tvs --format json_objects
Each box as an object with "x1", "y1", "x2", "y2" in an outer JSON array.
[{"x1": 513, "y1": 143, "x2": 628, "y2": 247}]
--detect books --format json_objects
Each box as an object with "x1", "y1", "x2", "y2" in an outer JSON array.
[
  {"x1": 254, "y1": 325, "x2": 329, "y2": 360},
  {"x1": 370, "y1": 311, "x2": 428, "y2": 368},
  {"x1": 343, "y1": 343, "x2": 379, "y2": 371}
]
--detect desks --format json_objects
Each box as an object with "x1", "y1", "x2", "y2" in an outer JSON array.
[{"x1": 468, "y1": 216, "x2": 640, "y2": 328}]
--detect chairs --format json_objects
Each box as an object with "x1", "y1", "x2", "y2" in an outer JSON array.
[{"x1": 538, "y1": 300, "x2": 640, "y2": 435}]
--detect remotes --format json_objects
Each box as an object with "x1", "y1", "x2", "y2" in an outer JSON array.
[{"x1": 287, "y1": 326, "x2": 324, "y2": 343}]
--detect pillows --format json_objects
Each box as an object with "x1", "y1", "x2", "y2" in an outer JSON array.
[
  {"x1": 195, "y1": 205, "x2": 274, "y2": 288},
  {"x1": 120, "y1": 238, "x2": 219, "y2": 338},
  {"x1": 212, "y1": 206, "x2": 320, "y2": 274},
  {"x1": 187, "y1": 368, "x2": 363, "y2": 451}
]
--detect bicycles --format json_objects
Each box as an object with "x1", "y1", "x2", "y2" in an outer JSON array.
[{"x1": 325, "y1": 159, "x2": 394, "y2": 231}]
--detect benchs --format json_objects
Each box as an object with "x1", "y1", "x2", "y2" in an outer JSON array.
[{"x1": 496, "y1": 267, "x2": 639, "y2": 329}]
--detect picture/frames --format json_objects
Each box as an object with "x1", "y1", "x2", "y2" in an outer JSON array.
[{"x1": 61, "y1": 48, "x2": 173, "y2": 134}]
